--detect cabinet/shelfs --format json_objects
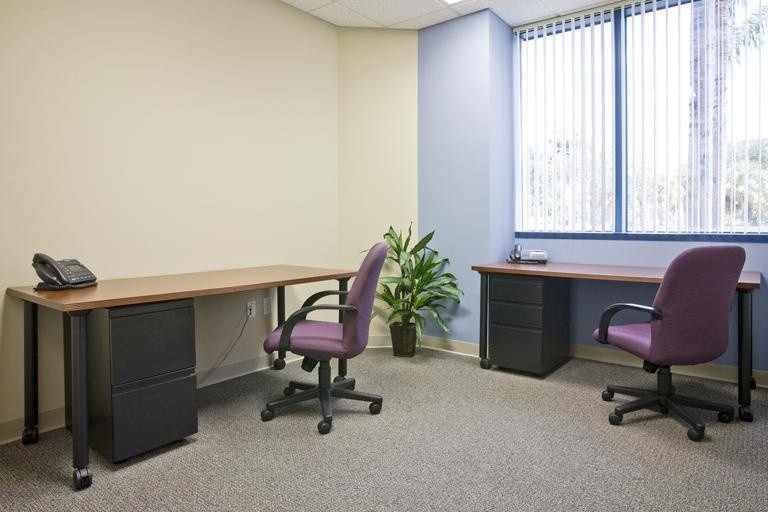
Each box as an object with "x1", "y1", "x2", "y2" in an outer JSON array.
[
  {"x1": 63, "y1": 298, "x2": 199, "y2": 464},
  {"x1": 488, "y1": 273, "x2": 569, "y2": 376}
]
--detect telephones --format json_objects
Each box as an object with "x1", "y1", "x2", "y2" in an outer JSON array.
[
  {"x1": 506, "y1": 242, "x2": 550, "y2": 264},
  {"x1": 31, "y1": 252, "x2": 98, "y2": 291}
]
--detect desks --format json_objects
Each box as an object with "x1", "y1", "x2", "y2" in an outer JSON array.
[
  {"x1": 5, "y1": 265, "x2": 358, "y2": 490},
  {"x1": 470, "y1": 260, "x2": 762, "y2": 423}
]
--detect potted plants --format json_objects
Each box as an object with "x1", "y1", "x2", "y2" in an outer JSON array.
[{"x1": 370, "y1": 221, "x2": 466, "y2": 357}]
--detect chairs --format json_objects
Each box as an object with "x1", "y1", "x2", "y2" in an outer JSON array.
[
  {"x1": 262, "y1": 242, "x2": 389, "y2": 434},
  {"x1": 594, "y1": 246, "x2": 749, "y2": 442}
]
